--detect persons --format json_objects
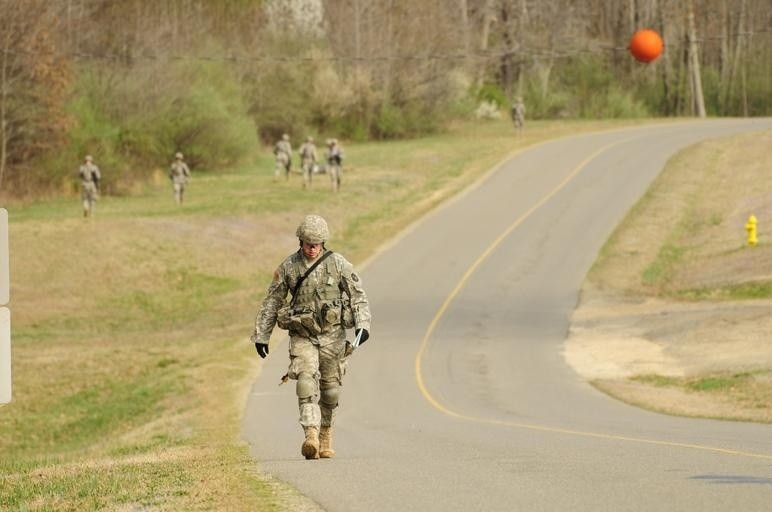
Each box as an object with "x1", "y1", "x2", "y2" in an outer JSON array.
[
  {"x1": 168, "y1": 151, "x2": 191, "y2": 202},
  {"x1": 273, "y1": 133, "x2": 293, "y2": 181},
  {"x1": 510, "y1": 96, "x2": 525, "y2": 138},
  {"x1": 78, "y1": 155, "x2": 101, "y2": 218},
  {"x1": 300, "y1": 137, "x2": 318, "y2": 188},
  {"x1": 323, "y1": 138, "x2": 344, "y2": 194},
  {"x1": 250, "y1": 213, "x2": 372, "y2": 462}
]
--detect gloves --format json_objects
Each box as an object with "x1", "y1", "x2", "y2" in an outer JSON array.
[
  {"x1": 355, "y1": 328, "x2": 369, "y2": 346},
  {"x1": 255, "y1": 343, "x2": 269, "y2": 358}
]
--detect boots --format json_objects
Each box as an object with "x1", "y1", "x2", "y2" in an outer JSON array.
[
  {"x1": 302, "y1": 424, "x2": 321, "y2": 460},
  {"x1": 319, "y1": 426, "x2": 336, "y2": 458}
]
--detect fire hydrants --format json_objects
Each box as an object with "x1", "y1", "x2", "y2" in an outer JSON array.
[{"x1": 744, "y1": 214, "x2": 759, "y2": 245}]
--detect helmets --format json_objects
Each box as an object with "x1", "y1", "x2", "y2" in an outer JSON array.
[{"x1": 296, "y1": 214, "x2": 329, "y2": 245}]
